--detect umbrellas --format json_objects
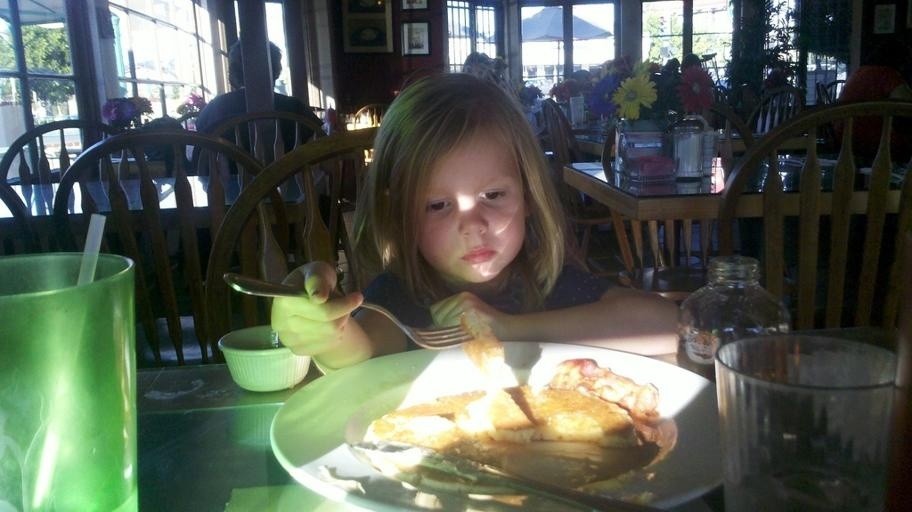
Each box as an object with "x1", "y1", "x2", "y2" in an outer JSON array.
[{"x1": 486, "y1": 6, "x2": 612, "y2": 84}]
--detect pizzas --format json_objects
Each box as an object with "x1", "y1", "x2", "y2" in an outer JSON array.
[
  {"x1": 369, "y1": 359, "x2": 657, "y2": 490},
  {"x1": 459, "y1": 309, "x2": 505, "y2": 372}
]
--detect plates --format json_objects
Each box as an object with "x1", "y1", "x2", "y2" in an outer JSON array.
[{"x1": 267, "y1": 338, "x2": 728, "y2": 511}]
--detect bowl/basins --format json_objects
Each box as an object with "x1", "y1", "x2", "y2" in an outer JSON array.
[{"x1": 218, "y1": 325, "x2": 310, "y2": 393}]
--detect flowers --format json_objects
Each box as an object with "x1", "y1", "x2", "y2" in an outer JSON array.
[
  {"x1": 675, "y1": 67, "x2": 714, "y2": 113},
  {"x1": 611, "y1": 73, "x2": 658, "y2": 120}
]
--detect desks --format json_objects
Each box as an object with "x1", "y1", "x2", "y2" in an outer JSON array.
[
  {"x1": 563, "y1": 152, "x2": 912, "y2": 327},
  {"x1": 1, "y1": 173, "x2": 301, "y2": 365},
  {"x1": 575, "y1": 130, "x2": 806, "y2": 269}
]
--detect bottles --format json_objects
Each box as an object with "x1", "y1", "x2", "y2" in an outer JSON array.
[
  {"x1": 667, "y1": 119, "x2": 706, "y2": 181},
  {"x1": 676, "y1": 252, "x2": 795, "y2": 375}
]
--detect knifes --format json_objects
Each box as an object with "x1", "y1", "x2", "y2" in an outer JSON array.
[{"x1": 347, "y1": 433, "x2": 663, "y2": 512}]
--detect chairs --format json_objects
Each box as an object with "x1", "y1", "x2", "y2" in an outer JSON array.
[
  {"x1": 0, "y1": 101, "x2": 403, "y2": 365},
  {"x1": 540, "y1": 81, "x2": 912, "y2": 328}
]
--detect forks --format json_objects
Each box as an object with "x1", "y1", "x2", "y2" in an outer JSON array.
[{"x1": 220, "y1": 267, "x2": 473, "y2": 351}]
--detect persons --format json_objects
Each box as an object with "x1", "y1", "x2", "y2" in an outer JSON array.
[
  {"x1": 269, "y1": 74, "x2": 684, "y2": 374},
  {"x1": 196, "y1": 38, "x2": 343, "y2": 245},
  {"x1": 834, "y1": 35, "x2": 912, "y2": 165}
]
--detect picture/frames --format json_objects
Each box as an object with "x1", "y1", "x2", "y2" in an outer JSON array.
[
  {"x1": 338, "y1": 0, "x2": 395, "y2": 55},
  {"x1": 399, "y1": 0, "x2": 430, "y2": 11},
  {"x1": 401, "y1": 21, "x2": 431, "y2": 57}
]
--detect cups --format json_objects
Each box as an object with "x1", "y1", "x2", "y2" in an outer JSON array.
[
  {"x1": 0, "y1": 251, "x2": 141, "y2": 512},
  {"x1": 569, "y1": 96, "x2": 585, "y2": 126},
  {"x1": 712, "y1": 331, "x2": 912, "y2": 512}
]
